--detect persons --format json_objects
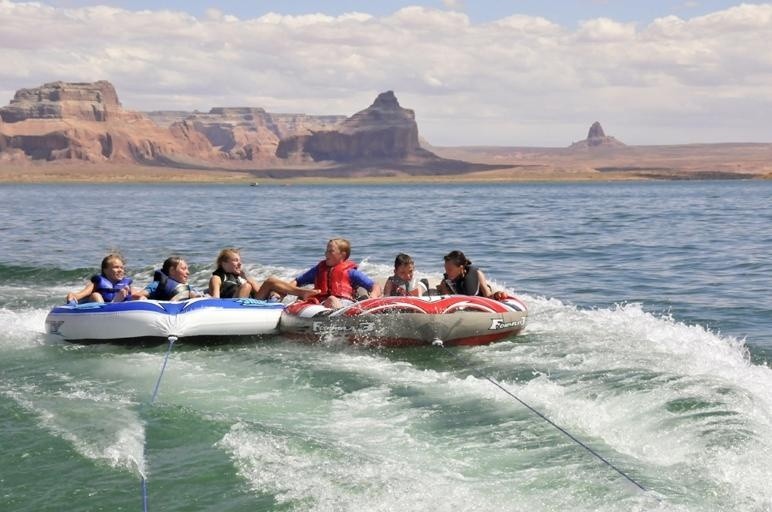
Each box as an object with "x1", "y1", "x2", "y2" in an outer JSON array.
[
  {"x1": 269, "y1": 239, "x2": 382, "y2": 304},
  {"x1": 438, "y1": 250, "x2": 508, "y2": 300},
  {"x1": 210, "y1": 249, "x2": 321, "y2": 301},
  {"x1": 132, "y1": 257, "x2": 212, "y2": 301},
  {"x1": 383, "y1": 254, "x2": 422, "y2": 297},
  {"x1": 67, "y1": 255, "x2": 147, "y2": 302}
]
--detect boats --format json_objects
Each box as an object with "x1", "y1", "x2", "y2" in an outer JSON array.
[
  {"x1": 280, "y1": 287, "x2": 530, "y2": 350},
  {"x1": 251, "y1": 182, "x2": 258, "y2": 188},
  {"x1": 43, "y1": 290, "x2": 292, "y2": 347}
]
